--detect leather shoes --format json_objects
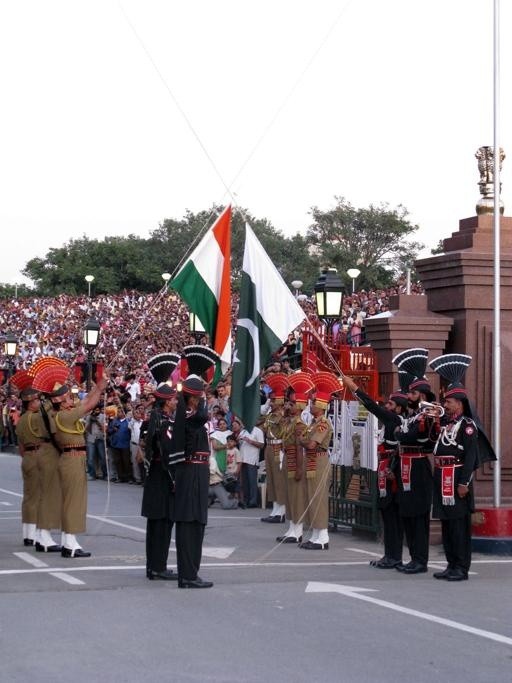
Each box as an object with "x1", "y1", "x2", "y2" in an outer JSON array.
[
  {"x1": 146, "y1": 566, "x2": 213, "y2": 587},
  {"x1": 371, "y1": 555, "x2": 468, "y2": 580}
]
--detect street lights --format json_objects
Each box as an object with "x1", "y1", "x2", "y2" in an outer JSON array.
[
  {"x1": 82, "y1": 310, "x2": 101, "y2": 389},
  {"x1": 347, "y1": 268, "x2": 362, "y2": 292},
  {"x1": 161, "y1": 272, "x2": 171, "y2": 293},
  {"x1": 12, "y1": 271, "x2": 22, "y2": 298},
  {"x1": 4, "y1": 332, "x2": 20, "y2": 445},
  {"x1": 291, "y1": 279, "x2": 303, "y2": 297},
  {"x1": 186, "y1": 305, "x2": 208, "y2": 345},
  {"x1": 314, "y1": 266, "x2": 344, "y2": 367},
  {"x1": 84, "y1": 274, "x2": 95, "y2": 296}
]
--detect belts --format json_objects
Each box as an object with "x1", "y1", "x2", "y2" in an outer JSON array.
[
  {"x1": 60, "y1": 446, "x2": 87, "y2": 452},
  {"x1": 26, "y1": 446, "x2": 40, "y2": 450}
]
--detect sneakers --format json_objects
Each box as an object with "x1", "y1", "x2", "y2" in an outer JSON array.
[
  {"x1": 261, "y1": 514, "x2": 329, "y2": 550},
  {"x1": 23, "y1": 539, "x2": 91, "y2": 557}
]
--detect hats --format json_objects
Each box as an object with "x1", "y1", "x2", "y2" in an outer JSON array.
[
  {"x1": 409, "y1": 379, "x2": 430, "y2": 393},
  {"x1": 443, "y1": 383, "x2": 465, "y2": 399},
  {"x1": 270, "y1": 392, "x2": 331, "y2": 403},
  {"x1": 390, "y1": 390, "x2": 408, "y2": 407},
  {"x1": 20, "y1": 385, "x2": 70, "y2": 403},
  {"x1": 156, "y1": 385, "x2": 176, "y2": 398},
  {"x1": 182, "y1": 378, "x2": 203, "y2": 395}
]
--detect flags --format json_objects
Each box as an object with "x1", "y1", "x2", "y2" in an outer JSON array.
[
  {"x1": 168, "y1": 203, "x2": 233, "y2": 392},
  {"x1": 229, "y1": 223, "x2": 306, "y2": 434}
]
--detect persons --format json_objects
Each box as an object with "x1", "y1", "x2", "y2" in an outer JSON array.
[
  {"x1": 212, "y1": 348, "x2": 436, "y2": 574},
  {"x1": 1, "y1": 292, "x2": 207, "y2": 487},
  {"x1": 9, "y1": 356, "x2": 109, "y2": 558},
  {"x1": 140, "y1": 345, "x2": 218, "y2": 588},
  {"x1": 428, "y1": 352, "x2": 497, "y2": 581},
  {"x1": 209, "y1": 281, "x2": 423, "y2": 512}
]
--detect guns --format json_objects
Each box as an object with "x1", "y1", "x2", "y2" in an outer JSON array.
[
  {"x1": 155, "y1": 403, "x2": 176, "y2": 495},
  {"x1": 36, "y1": 394, "x2": 56, "y2": 447}
]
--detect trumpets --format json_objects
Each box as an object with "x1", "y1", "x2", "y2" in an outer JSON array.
[{"x1": 419, "y1": 400, "x2": 446, "y2": 417}]
